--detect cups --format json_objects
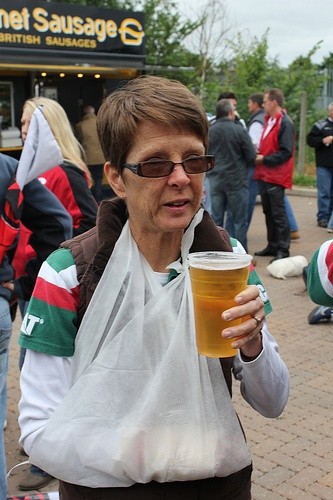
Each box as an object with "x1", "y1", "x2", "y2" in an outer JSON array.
[{"x1": 186, "y1": 251, "x2": 254, "y2": 358}]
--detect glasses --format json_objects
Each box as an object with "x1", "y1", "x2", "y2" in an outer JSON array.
[{"x1": 122, "y1": 155, "x2": 215, "y2": 178}]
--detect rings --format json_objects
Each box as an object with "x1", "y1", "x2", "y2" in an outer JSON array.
[{"x1": 251, "y1": 316, "x2": 262, "y2": 326}]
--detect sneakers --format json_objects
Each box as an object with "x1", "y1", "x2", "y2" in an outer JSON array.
[
  {"x1": 20, "y1": 447, "x2": 29, "y2": 457},
  {"x1": 18, "y1": 472, "x2": 52, "y2": 491}
]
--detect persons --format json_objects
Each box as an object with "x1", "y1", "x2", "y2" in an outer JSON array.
[
  {"x1": 0, "y1": 145, "x2": 73, "y2": 500},
  {"x1": 17, "y1": 76, "x2": 290, "y2": 500},
  {"x1": 246, "y1": 93, "x2": 302, "y2": 239},
  {"x1": 254, "y1": 88, "x2": 296, "y2": 264},
  {"x1": 203, "y1": 92, "x2": 248, "y2": 213},
  {"x1": 306, "y1": 101, "x2": 333, "y2": 228},
  {"x1": 206, "y1": 99, "x2": 258, "y2": 254},
  {"x1": 11, "y1": 95, "x2": 99, "y2": 490},
  {"x1": 74, "y1": 104, "x2": 107, "y2": 204}
]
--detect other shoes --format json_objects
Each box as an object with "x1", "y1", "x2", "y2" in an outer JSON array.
[
  {"x1": 290, "y1": 231, "x2": 300, "y2": 239},
  {"x1": 4, "y1": 419, "x2": 7, "y2": 429},
  {"x1": 328, "y1": 229, "x2": 333, "y2": 233},
  {"x1": 303, "y1": 266, "x2": 308, "y2": 284},
  {"x1": 318, "y1": 222, "x2": 328, "y2": 227},
  {"x1": 251, "y1": 259, "x2": 256, "y2": 267},
  {"x1": 308, "y1": 305, "x2": 333, "y2": 324},
  {"x1": 270, "y1": 251, "x2": 289, "y2": 263},
  {"x1": 254, "y1": 246, "x2": 278, "y2": 256}
]
